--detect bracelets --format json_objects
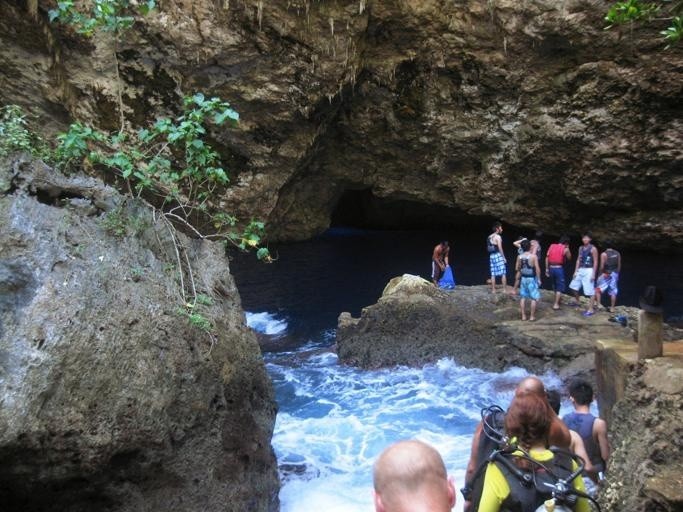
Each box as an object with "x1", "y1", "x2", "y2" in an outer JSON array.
[{"x1": 545, "y1": 268, "x2": 549, "y2": 273}]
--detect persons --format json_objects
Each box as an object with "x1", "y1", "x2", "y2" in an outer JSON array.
[
  {"x1": 568, "y1": 232, "x2": 598, "y2": 315},
  {"x1": 515, "y1": 240, "x2": 542, "y2": 321},
  {"x1": 464, "y1": 378, "x2": 571, "y2": 512},
  {"x1": 374, "y1": 440, "x2": 456, "y2": 512},
  {"x1": 508, "y1": 233, "x2": 529, "y2": 294},
  {"x1": 528, "y1": 240, "x2": 541, "y2": 302},
  {"x1": 431, "y1": 240, "x2": 449, "y2": 287},
  {"x1": 546, "y1": 391, "x2": 593, "y2": 472},
  {"x1": 486, "y1": 222, "x2": 508, "y2": 293},
  {"x1": 561, "y1": 380, "x2": 609, "y2": 483},
  {"x1": 477, "y1": 392, "x2": 591, "y2": 512},
  {"x1": 595, "y1": 242, "x2": 621, "y2": 312},
  {"x1": 545, "y1": 235, "x2": 572, "y2": 309}
]
